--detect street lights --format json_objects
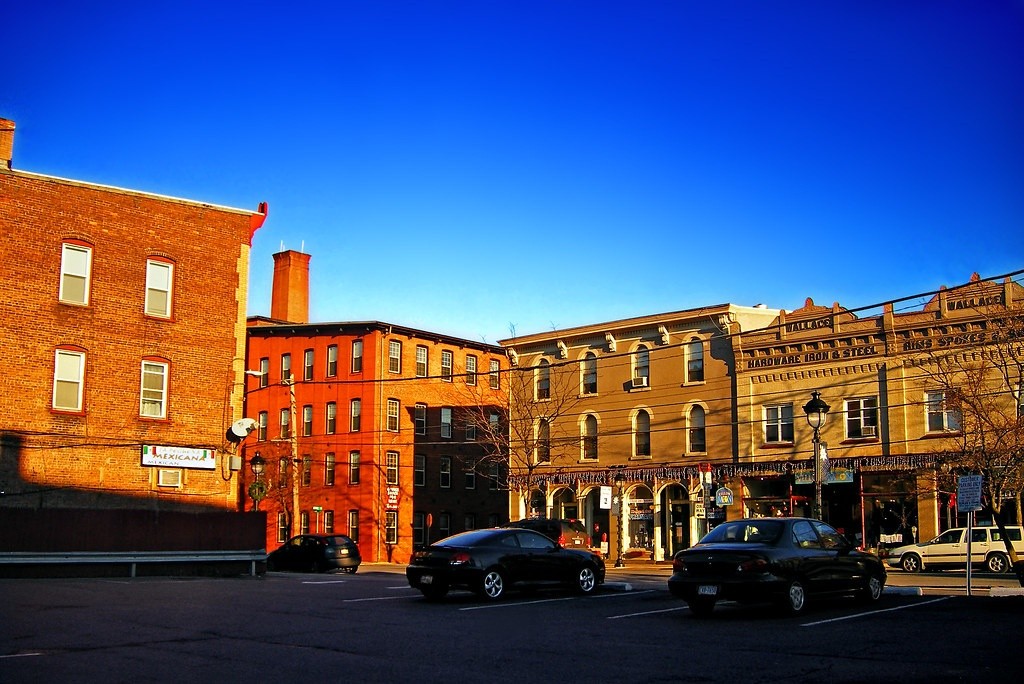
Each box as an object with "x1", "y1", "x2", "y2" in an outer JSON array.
[
  {"x1": 614, "y1": 470, "x2": 627, "y2": 567},
  {"x1": 803, "y1": 391, "x2": 831, "y2": 517},
  {"x1": 250, "y1": 449, "x2": 264, "y2": 511}
]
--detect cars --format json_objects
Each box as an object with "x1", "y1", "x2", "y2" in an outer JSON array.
[
  {"x1": 267, "y1": 533, "x2": 362, "y2": 576},
  {"x1": 667, "y1": 517, "x2": 888, "y2": 615},
  {"x1": 406, "y1": 525, "x2": 607, "y2": 601}
]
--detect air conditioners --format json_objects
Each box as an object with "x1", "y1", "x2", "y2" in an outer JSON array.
[
  {"x1": 632, "y1": 376, "x2": 648, "y2": 387},
  {"x1": 862, "y1": 426, "x2": 876, "y2": 436}
]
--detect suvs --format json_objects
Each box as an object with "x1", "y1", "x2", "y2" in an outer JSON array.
[
  {"x1": 502, "y1": 518, "x2": 592, "y2": 551},
  {"x1": 888, "y1": 525, "x2": 1023, "y2": 573}
]
